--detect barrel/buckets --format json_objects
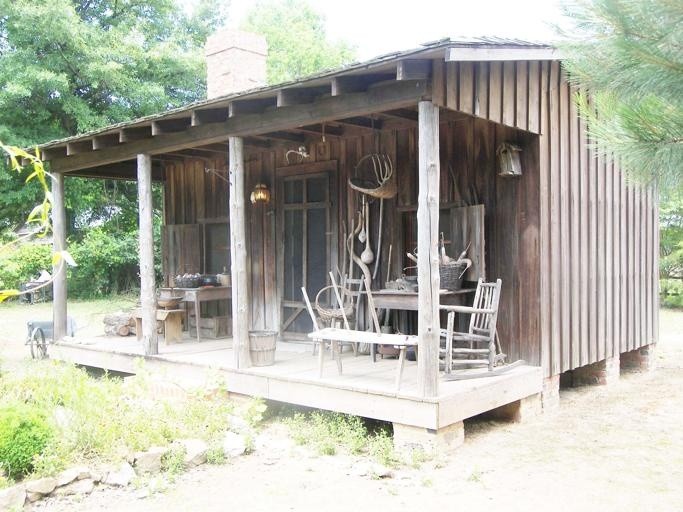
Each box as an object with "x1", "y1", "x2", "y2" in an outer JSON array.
[{"x1": 248, "y1": 330, "x2": 278, "y2": 366}]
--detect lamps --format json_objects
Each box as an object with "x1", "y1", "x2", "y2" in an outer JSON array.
[{"x1": 250, "y1": 150, "x2": 271, "y2": 208}]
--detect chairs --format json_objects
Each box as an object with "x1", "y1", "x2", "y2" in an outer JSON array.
[{"x1": 438, "y1": 278, "x2": 503, "y2": 374}]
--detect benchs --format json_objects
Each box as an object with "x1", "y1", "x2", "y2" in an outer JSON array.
[
  {"x1": 134, "y1": 308, "x2": 187, "y2": 345},
  {"x1": 309, "y1": 328, "x2": 420, "y2": 383}
]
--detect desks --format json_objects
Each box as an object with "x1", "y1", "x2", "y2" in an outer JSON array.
[
  {"x1": 360, "y1": 287, "x2": 478, "y2": 364},
  {"x1": 21, "y1": 281, "x2": 54, "y2": 305},
  {"x1": 158, "y1": 285, "x2": 234, "y2": 344}
]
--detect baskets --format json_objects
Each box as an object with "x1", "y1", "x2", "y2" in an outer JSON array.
[
  {"x1": 157, "y1": 286, "x2": 183, "y2": 308},
  {"x1": 315, "y1": 285, "x2": 354, "y2": 321},
  {"x1": 414, "y1": 261, "x2": 467, "y2": 292},
  {"x1": 346, "y1": 151, "x2": 399, "y2": 201}
]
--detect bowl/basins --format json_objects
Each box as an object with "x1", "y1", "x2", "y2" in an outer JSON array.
[{"x1": 158, "y1": 296, "x2": 182, "y2": 308}]
